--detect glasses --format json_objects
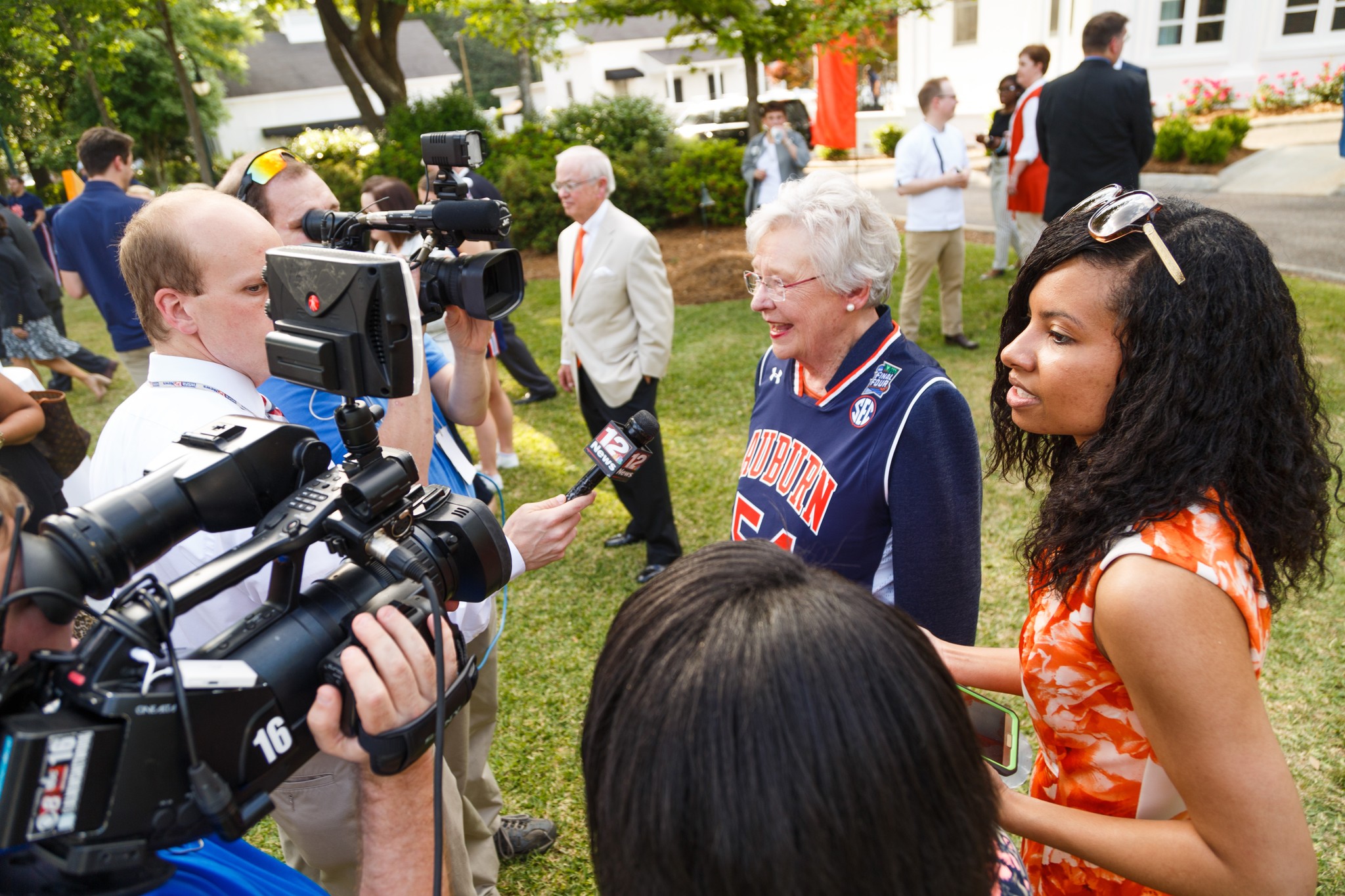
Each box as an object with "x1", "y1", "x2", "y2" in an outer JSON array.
[
  {"x1": 550, "y1": 177, "x2": 598, "y2": 194},
  {"x1": 744, "y1": 270, "x2": 825, "y2": 301},
  {"x1": 237, "y1": 147, "x2": 306, "y2": 201},
  {"x1": 1058, "y1": 184, "x2": 1185, "y2": 285}
]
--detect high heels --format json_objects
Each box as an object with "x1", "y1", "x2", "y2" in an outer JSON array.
[
  {"x1": 86, "y1": 373, "x2": 113, "y2": 401},
  {"x1": 945, "y1": 333, "x2": 979, "y2": 349}
]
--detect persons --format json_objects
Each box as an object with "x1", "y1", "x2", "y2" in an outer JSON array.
[
  {"x1": 578, "y1": 541, "x2": 1031, "y2": 896},
  {"x1": 866, "y1": 59, "x2": 881, "y2": 111},
  {"x1": 764, "y1": 60, "x2": 791, "y2": 94},
  {"x1": 0, "y1": 127, "x2": 598, "y2": 896},
  {"x1": 743, "y1": 101, "x2": 812, "y2": 217},
  {"x1": 891, "y1": 11, "x2": 1157, "y2": 350},
  {"x1": 549, "y1": 144, "x2": 685, "y2": 584},
  {"x1": 727, "y1": 171, "x2": 981, "y2": 650},
  {"x1": 913, "y1": 193, "x2": 1345, "y2": 896}
]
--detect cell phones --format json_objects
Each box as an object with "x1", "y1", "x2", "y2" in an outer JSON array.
[{"x1": 954, "y1": 683, "x2": 1021, "y2": 777}]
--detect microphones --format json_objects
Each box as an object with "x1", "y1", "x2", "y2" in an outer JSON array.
[
  {"x1": 415, "y1": 200, "x2": 501, "y2": 233},
  {"x1": 565, "y1": 410, "x2": 660, "y2": 501}
]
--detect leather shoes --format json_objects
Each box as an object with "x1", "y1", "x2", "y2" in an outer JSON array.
[
  {"x1": 638, "y1": 564, "x2": 665, "y2": 584},
  {"x1": 605, "y1": 531, "x2": 641, "y2": 547}
]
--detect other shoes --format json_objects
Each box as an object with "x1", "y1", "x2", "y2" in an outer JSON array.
[
  {"x1": 512, "y1": 384, "x2": 557, "y2": 404},
  {"x1": 89, "y1": 356, "x2": 118, "y2": 380},
  {"x1": 980, "y1": 268, "x2": 1004, "y2": 279},
  {"x1": 47, "y1": 373, "x2": 74, "y2": 391}
]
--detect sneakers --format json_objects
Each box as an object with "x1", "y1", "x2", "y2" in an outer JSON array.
[
  {"x1": 480, "y1": 473, "x2": 504, "y2": 492},
  {"x1": 493, "y1": 813, "x2": 556, "y2": 862},
  {"x1": 473, "y1": 449, "x2": 518, "y2": 470}
]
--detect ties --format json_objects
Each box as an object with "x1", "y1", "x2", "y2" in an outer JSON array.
[{"x1": 572, "y1": 226, "x2": 587, "y2": 367}]
